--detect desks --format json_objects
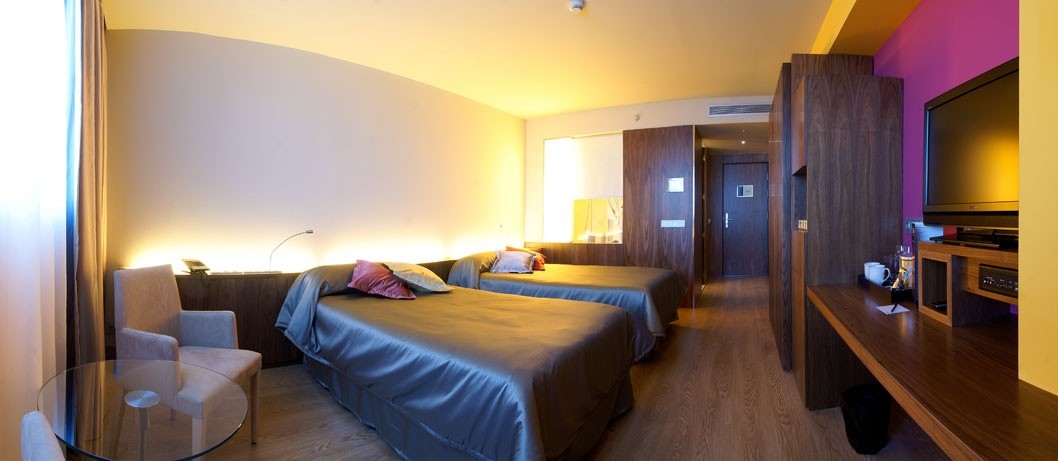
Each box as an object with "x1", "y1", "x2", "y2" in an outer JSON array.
[
  {"x1": 916, "y1": 241, "x2": 1019, "y2": 328},
  {"x1": 807, "y1": 276, "x2": 1058, "y2": 461}
]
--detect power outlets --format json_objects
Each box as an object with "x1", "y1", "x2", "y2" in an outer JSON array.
[{"x1": 906, "y1": 218, "x2": 923, "y2": 233}]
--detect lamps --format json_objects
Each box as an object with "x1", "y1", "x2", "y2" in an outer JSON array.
[{"x1": 269, "y1": 229, "x2": 314, "y2": 273}]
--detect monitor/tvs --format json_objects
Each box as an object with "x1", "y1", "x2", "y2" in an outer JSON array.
[{"x1": 922, "y1": 56, "x2": 1020, "y2": 250}]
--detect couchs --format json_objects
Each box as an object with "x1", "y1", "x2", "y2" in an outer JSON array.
[{"x1": 113, "y1": 264, "x2": 262, "y2": 461}]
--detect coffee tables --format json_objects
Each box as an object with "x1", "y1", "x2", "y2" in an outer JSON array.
[{"x1": 37, "y1": 360, "x2": 249, "y2": 461}]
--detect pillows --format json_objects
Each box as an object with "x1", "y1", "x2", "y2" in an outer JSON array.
[
  {"x1": 490, "y1": 251, "x2": 534, "y2": 274},
  {"x1": 506, "y1": 246, "x2": 547, "y2": 271},
  {"x1": 384, "y1": 261, "x2": 453, "y2": 294},
  {"x1": 346, "y1": 259, "x2": 417, "y2": 300}
]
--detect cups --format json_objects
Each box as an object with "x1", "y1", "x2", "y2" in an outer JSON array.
[{"x1": 863, "y1": 262, "x2": 891, "y2": 287}]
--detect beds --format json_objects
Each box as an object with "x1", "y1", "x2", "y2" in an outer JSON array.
[
  {"x1": 455, "y1": 251, "x2": 684, "y2": 362},
  {"x1": 273, "y1": 263, "x2": 636, "y2": 461}
]
